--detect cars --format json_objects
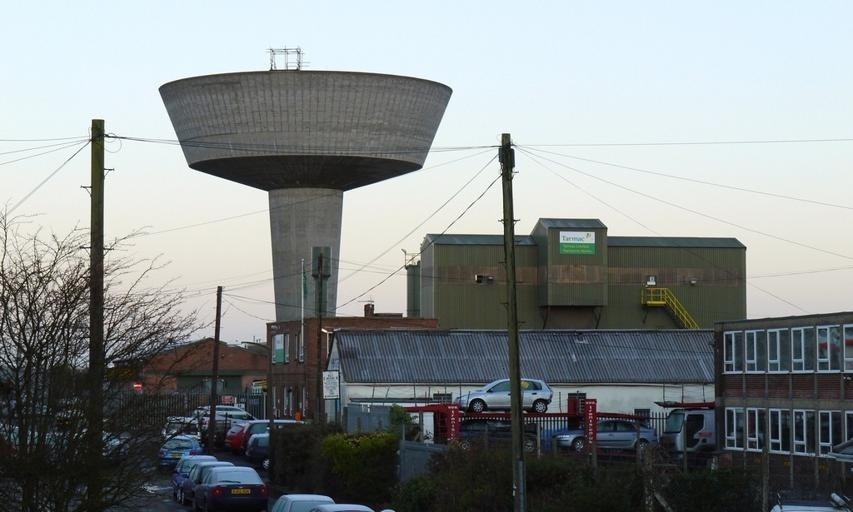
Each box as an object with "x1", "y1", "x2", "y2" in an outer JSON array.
[
  {"x1": 241, "y1": 415, "x2": 314, "y2": 473},
  {"x1": 552, "y1": 417, "x2": 660, "y2": 456},
  {"x1": 271, "y1": 493, "x2": 374, "y2": 512},
  {"x1": 457, "y1": 418, "x2": 545, "y2": 457},
  {"x1": 454, "y1": 378, "x2": 554, "y2": 413},
  {"x1": 155, "y1": 404, "x2": 270, "y2": 510},
  {"x1": 0, "y1": 411, "x2": 133, "y2": 470}
]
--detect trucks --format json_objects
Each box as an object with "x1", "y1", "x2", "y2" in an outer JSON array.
[{"x1": 660, "y1": 407, "x2": 720, "y2": 476}]
